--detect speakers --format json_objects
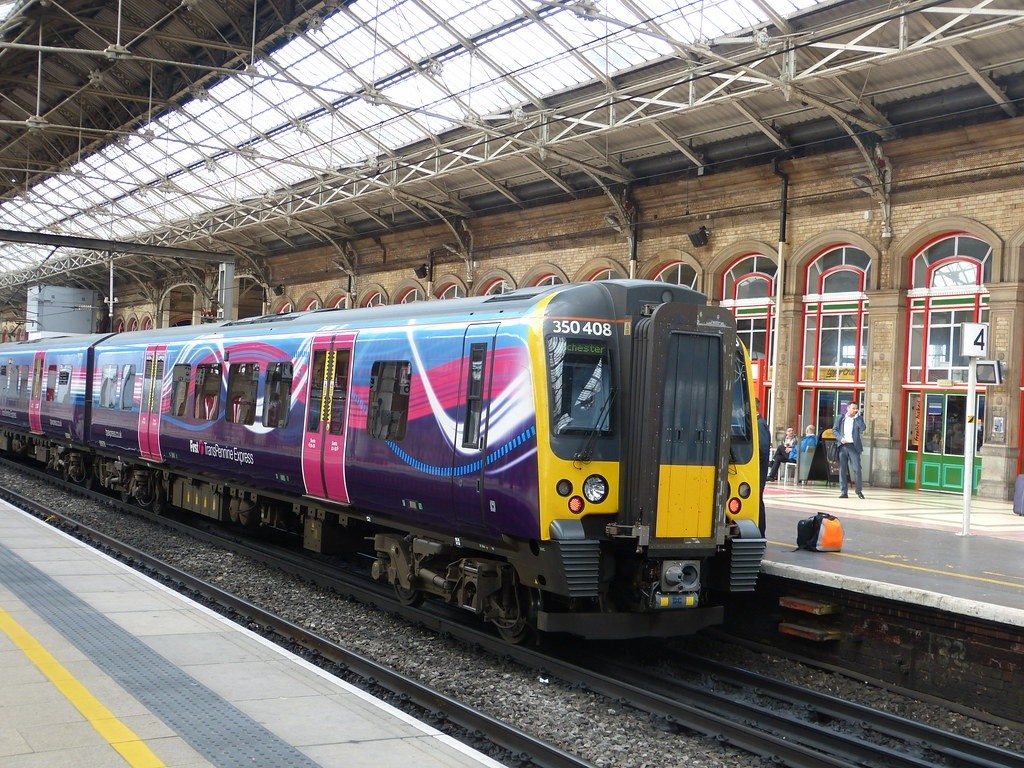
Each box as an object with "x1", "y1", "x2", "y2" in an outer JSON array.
[
  {"x1": 414, "y1": 265, "x2": 427, "y2": 278},
  {"x1": 688, "y1": 229, "x2": 708, "y2": 247},
  {"x1": 273, "y1": 286, "x2": 283, "y2": 296}
]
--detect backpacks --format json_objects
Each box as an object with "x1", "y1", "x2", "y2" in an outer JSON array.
[{"x1": 812, "y1": 512, "x2": 843, "y2": 552}]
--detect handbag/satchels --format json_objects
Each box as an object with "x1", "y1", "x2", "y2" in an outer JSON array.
[
  {"x1": 797, "y1": 515, "x2": 812, "y2": 547},
  {"x1": 827, "y1": 444, "x2": 839, "y2": 464}
]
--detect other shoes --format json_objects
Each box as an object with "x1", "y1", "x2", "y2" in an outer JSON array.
[
  {"x1": 765, "y1": 476, "x2": 777, "y2": 481},
  {"x1": 856, "y1": 488, "x2": 865, "y2": 499},
  {"x1": 839, "y1": 494, "x2": 848, "y2": 498}
]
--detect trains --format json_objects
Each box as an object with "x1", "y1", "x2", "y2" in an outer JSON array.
[{"x1": 0, "y1": 279, "x2": 769, "y2": 645}]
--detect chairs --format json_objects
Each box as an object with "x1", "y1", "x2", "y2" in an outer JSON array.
[{"x1": 767, "y1": 446, "x2": 816, "y2": 485}]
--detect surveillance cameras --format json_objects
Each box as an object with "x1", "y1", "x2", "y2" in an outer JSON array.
[
  {"x1": 104, "y1": 297, "x2": 109, "y2": 303},
  {"x1": 114, "y1": 297, "x2": 119, "y2": 303}
]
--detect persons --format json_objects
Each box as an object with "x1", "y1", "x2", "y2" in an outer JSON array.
[
  {"x1": 755, "y1": 398, "x2": 771, "y2": 538},
  {"x1": 927, "y1": 422, "x2": 963, "y2": 452},
  {"x1": 832, "y1": 402, "x2": 866, "y2": 499},
  {"x1": 766, "y1": 425, "x2": 817, "y2": 482},
  {"x1": 780, "y1": 427, "x2": 799, "y2": 447}
]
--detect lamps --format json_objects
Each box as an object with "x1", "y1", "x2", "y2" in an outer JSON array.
[
  {"x1": 332, "y1": 259, "x2": 346, "y2": 272},
  {"x1": 414, "y1": 263, "x2": 429, "y2": 279},
  {"x1": 605, "y1": 215, "x2": 622, "y2": 231},
  {"x1": 852, "y1": 176, "x2": 878, "y2": 197},
  {"x1": 273, "y1": 284, "x2": 285, "y2": 295},
  {"x1": 688, "y1": 226, "x2": 712, "y2": 247},
  {"x1": 442, "y1": 243, "x2": 460, "y2": 255},
  {"x1": 251, "y1": 274, "x2": 262, "y2": 284}
]
